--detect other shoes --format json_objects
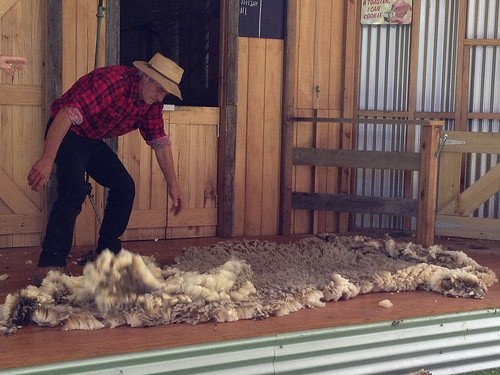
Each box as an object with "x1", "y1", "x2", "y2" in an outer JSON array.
[{"x1": 34, "y1": 267, "x2": 71, "y2": 279}]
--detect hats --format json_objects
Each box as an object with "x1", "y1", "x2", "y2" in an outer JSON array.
[{"x1": 133, "y1": 52, "x2": 184, "y2": 101}]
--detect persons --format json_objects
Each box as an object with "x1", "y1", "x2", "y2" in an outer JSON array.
[{"x1": 27, "y1": 53, "x2": 185, "y2": 278}]
